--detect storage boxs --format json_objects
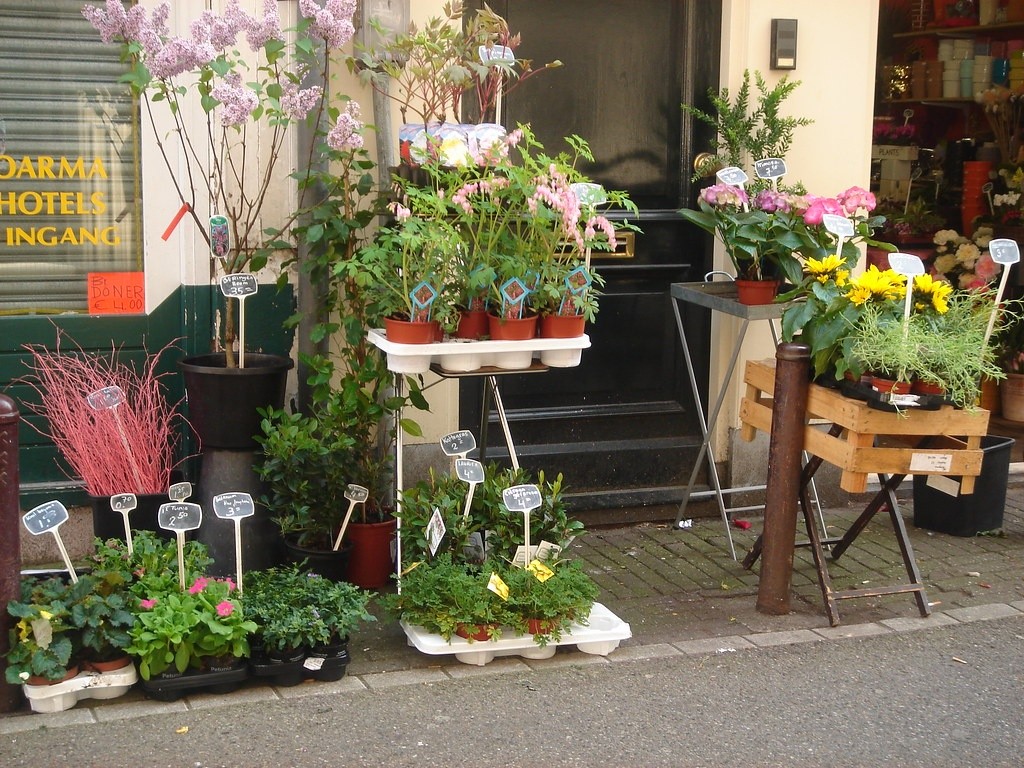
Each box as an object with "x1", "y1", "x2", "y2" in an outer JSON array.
[{"x1": 912, "y1": 433, "x2": 1015, "y2": 537}]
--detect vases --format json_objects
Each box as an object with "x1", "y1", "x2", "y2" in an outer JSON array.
[
  {"x1": 450, "y1": 310, "x2": 489, "y2": 341},
  {"x1": 200, "y1": 652, "x2": 241, "y2": 671},
  {"x1": 735, "y1": 278, "x2": 780, "y2": 305},
  {"x1": 999, "y1": 372, "x2": 1024, "y2": 421},
  {"x1": 1004, "y1": 225, "x2": 1024, "y2": 246},
  {"x1": 979, "y1": 373, "x2": 999, "y2": 417},
  {"x1": 383, "y1": 316, "x2": 439, "y2": 345},
  {"x1": 148, "y1": 666, "x2": 182, "y2": 680},
  {"x1": 486, "y1": 307, "x2": 538, "y2": 340},
  {"x1": 871, "y1": 144, "x2": 919, "y2": 160},
  {"x1": 178, "y1": 352, "x2": 295, "y2": 451},
  {"x1": 536, "y1": 310, "x2": 585, "y2": 338},
  {"x1": 432, "y1": 319, "x2": 445, "y2": 342}
]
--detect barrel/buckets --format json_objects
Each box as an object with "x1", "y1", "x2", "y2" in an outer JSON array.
[{"x1": 911, "y1": 432, "x2": 1016, "y2": 538}]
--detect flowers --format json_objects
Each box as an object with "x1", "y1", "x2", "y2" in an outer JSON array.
[
  {"x1": 994, "y1": 165, "x2": 1024, "y2": 226},
  {"x1": 76, "y1": 0, "x2": 364, "y2": 370},
  {"x1": 999, "y1": 343, "x2": 1024, "y2": 373},
  {"x1": 679, "y1": 183, "x2": 898, "y2": 284},
  {"x1": 82, "y1": 528, "x2": 254, "y2": 676},
  {"x1": 974, "y1": 82, "x2": 1024, "y2": 164},
  {"x1": 923, "y1": 222, "x2": 1005, "y2": 306},
  {"x1": 872, "y1": 122, "x2": 917, "y2": 145},
  {"x1": 329, "y1": 121, "x2": 644, "y2": 322},
  {"x1": 776, "y1": 255, "x2": 954, "y2": 382}
]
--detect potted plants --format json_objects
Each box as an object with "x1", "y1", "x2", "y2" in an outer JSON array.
[
  {"x1": 228, "y1": 93, "x2": 600, "y2": 664},
  {"x1": 338, "y1": 0, "x2": 565, "y2": 166},
  {"x1": 0, "y1": 567, "x2": 94, "y2": 686},
  {"x1": 834, "y1": 278, "x2": 1024, "y2": 420},
  {"x1": 869, "y1": 195, "x2": 949, "y2": 247},
  {"x1": 63, "y1": 571, "x2": 135, "y2": 671}
]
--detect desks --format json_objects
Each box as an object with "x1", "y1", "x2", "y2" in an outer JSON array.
[
  {"x1": 669, "y1": 275, "x2": 834, "y2": 564},
  {"x1": 742, "y1": 423, "x2": 940, "y2": 626}
]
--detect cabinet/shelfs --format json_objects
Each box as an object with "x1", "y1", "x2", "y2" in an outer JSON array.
[{"x1": 888, "y1": 21, "x2": 1024, "y2": 138}]
